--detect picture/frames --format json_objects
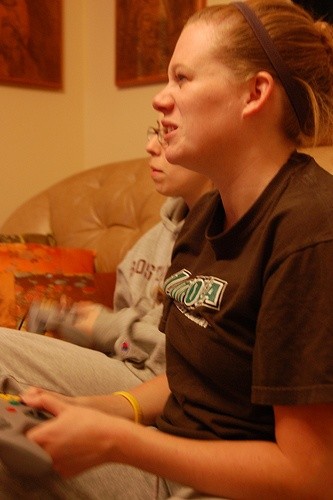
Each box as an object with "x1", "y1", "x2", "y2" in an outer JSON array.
[
  {"x1": 115, "y1": 0, "x2": 207, "y2": 88},
  {"x1": 0, "y1": 0, "x2": 64, "y2": 92}
]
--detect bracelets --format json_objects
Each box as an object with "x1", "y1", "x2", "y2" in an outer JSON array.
[{"x1": 113, "y1": 392, "x2": 142, "y2": 424}]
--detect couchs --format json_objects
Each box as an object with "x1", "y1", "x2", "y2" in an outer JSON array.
[{"x1": 0, "y1": 146, "x2": 333, "y2": 272}]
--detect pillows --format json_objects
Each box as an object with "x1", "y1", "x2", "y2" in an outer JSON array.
[{"x1": 0, "y1": 233, "x2": 116, "y2": 341}]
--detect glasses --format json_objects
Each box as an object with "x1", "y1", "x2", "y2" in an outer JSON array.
[{"x1": 146, "y1": 126, "x2": 169, "y2": 145}]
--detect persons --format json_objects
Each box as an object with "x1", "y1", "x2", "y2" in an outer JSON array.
[
  {"x1": 1, "y1": 113, "x2": 213, "y2": 396},
  {"x1": 21, "y1": 1, "x2": 333, "y2": 499}
]
